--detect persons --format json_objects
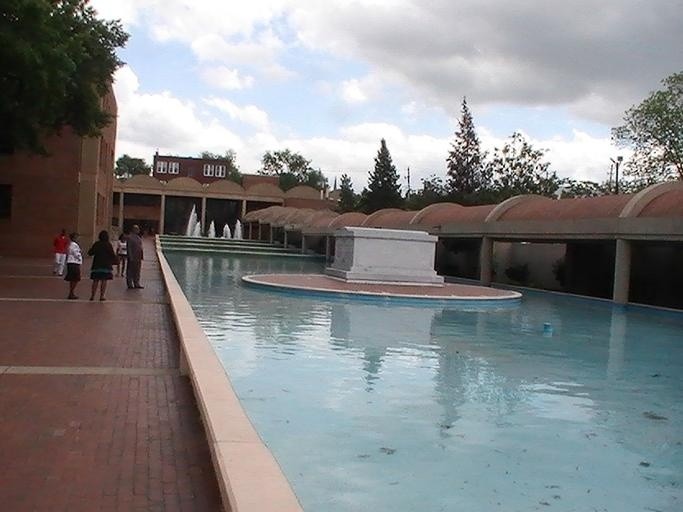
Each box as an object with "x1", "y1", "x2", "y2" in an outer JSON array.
[
  {"x1": 126, "y1": 224, "x2": 144, "y2": 288},
  {"x1": 115, "y1": 233, "x2": 126, "y2": 275},
  {"x1": 87, "y1": 230, "x2": 114, "y2": 301},
  {"x1": 51, "y1": 228, "x2": 67, "y2": 276},
  {"x1": 64, "y1": 232, "x2": 82, "y2": 299}
]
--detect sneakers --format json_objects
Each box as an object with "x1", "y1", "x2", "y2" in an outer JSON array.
[
  {"x1": 67, "y1": 294, "x2": 107, "y2": 301},
  {"x1": 127, "y1": 285, "x2": 146, "y2": 290}
]
